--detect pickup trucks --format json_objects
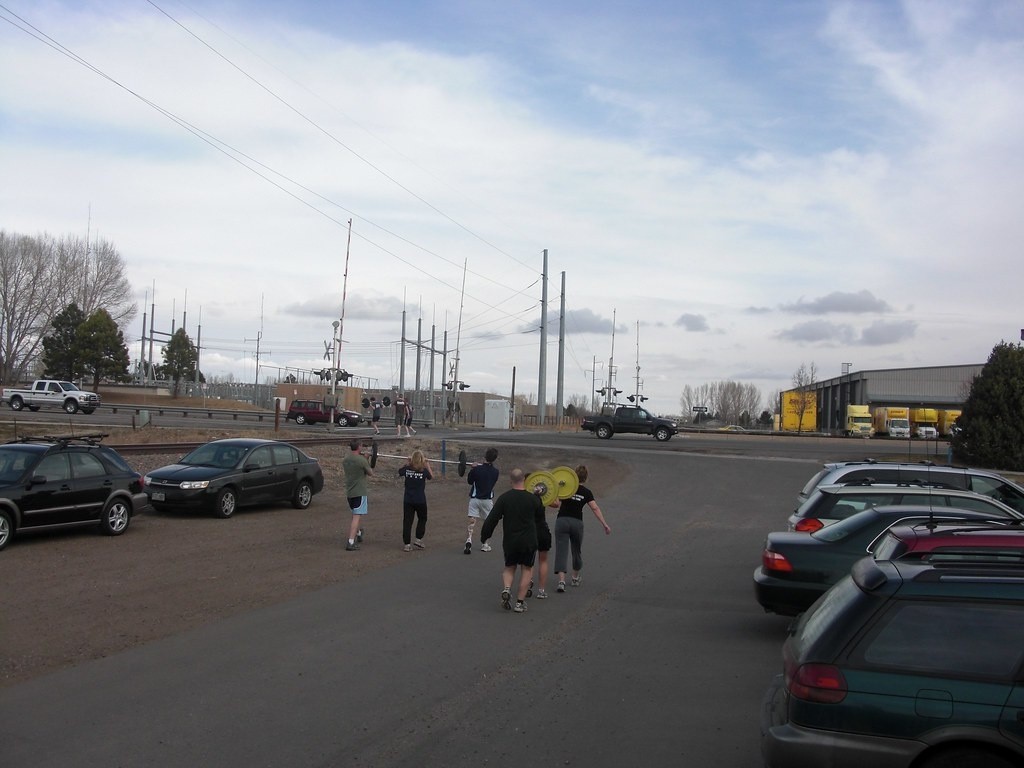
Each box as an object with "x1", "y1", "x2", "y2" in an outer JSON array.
[
  {"x1": 1, "y1": 380, "x2": 102, "y2": 415},
  {"x1": 580, "y1": 407, "x2": 679, "y2": 442}
]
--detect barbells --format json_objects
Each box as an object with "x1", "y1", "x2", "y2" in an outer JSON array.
[
  {"x1": 525, "y1": 464, "x2": 579, "y2": 507},
  {"x1": 359, "y1": 442, "x2": 482, "y2": 477},
  {"x1": 361, "y1": 396, "x2": 392, "y2": 408}
]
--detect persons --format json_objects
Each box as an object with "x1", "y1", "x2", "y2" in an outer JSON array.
[
  {"x1": 370, "y1": 397, "x2": 384, "y2": 435},
  {"x1": 463, "y1": 448, "x2": 499, "y2": 554},
  {"x1": 342, "y1": 439, "x2": 373, "y2": 551},
  {"x1": 394, "y1": 394, "x2": 416, "y2": 438},
  {"x1": 481, "y1": 465, "x2": 611, "y2": 612},
  {"x1": 398, "y1": 451, "x2": 434, "y2": 551}
]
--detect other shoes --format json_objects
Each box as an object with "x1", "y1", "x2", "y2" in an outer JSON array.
[
  {"x1": 412, "y1": 430, "x2": 416, "y2": 436},
  {"x1": 404, "y1": 435, "x2": 410, "y2": 437}
]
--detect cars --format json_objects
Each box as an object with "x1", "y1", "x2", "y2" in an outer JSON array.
[
  {"x1": 141, "y1": 436, "x2": 325, "y2": 519},
  {"x1": 718, "y1": 424, "x2": 745, "y2": 431},
  {"x1": 753, "y1": 456, "x2": 1024, "y2": 619}
]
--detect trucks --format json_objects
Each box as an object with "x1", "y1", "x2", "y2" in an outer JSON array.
[
  {"x1": 909, "y1": 407, "x2": 940, "y2": 442},
  {"x1": 938, "y1": 409, "x2": 963, "y2": 440},
  {"x1": 843, "y1": 404, "x2": 875, "y2": 439},
  {"x1": 872, "y1": 406, "x2": 910, "y2": 440}
]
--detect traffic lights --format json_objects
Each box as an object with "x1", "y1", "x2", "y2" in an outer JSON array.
[
  {"x1": 342, "y1": 372, "x2": 353, "y2": 381},
  {"x1": 595, "y1": 389, "x2": 606, "y2": 396},
  {"x1": 613, "y1": 390, "x2": 623, "y2": 396},
  {"x1": 442, "y1": 383, "x2": 453, "y2": 390},
  {"x1": 314, "y1": 370, "x2": 326, "y2": 381},
  {"x1": 459, "y1": 384, "x2": 470, "y2": 390},
  {"x1": 640, "y1": 397, "x2": 648, "y2": 402},
  {"x1": 627, "y1": 396, "x2": 635, "y2": 402}
]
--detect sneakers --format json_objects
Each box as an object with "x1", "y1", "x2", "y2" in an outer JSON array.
[
  {"x1": 346, "y1": 541, "x2": 360, "y2": 550},
  {"x1": 537, "y1": 591, "x2": 548, "y2": 598},
  {"x1": 404, "y1": 545, "x2": 413, "y2": 552},
  {"x1": 501, "y1": 589, "x2": 512, "y2": 609},
  {"x1": 514, "y1": 602, "x2": 528, "y2": 612},
  {"x1": 571, "y1": 576, "x2": 580, "y2": 586},
  {"x1": 557, "y1": 581, "x2": 566, "y2": 592},
  {"x1": 357, "y1": 529, "x2": 364, "y2": 542},
  {"x1": 526, "y1": 580, "x2": 533, "y2": 598},
  {"x1": 480, "y1": 545, "x2": 491, "y2": 552},
  {"x1": 414, "y1": 539, "x2": 425, "y2": 548},
  {"x1": 464, "y1": 539, "x2": 472, "y2": 554}
]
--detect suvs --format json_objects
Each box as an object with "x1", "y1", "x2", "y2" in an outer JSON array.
[
  {"x1": 286, "y1": 398, "x2": 365, "y2": 427},
  {"x1": 0, "y1": 432, "x2": 149, "y2": 552},
  {"x1": 757, "y1": 546, "x2": 1024, "y2": 768}
]
